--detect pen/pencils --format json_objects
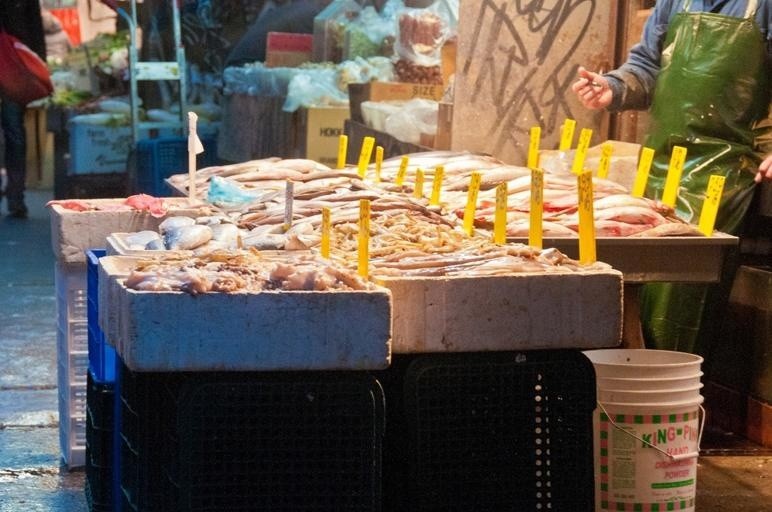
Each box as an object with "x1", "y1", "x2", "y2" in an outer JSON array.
[{"x1": 578, "y1": 78, "x2": 602, "y2": 88}]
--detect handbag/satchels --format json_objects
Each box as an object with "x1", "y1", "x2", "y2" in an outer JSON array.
[{"x1": 0, "y1": 32, "x2": 52, "y2": 105}]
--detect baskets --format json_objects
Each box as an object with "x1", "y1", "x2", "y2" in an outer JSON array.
[
  {"x1": 135, "y1": 136, "x2": 216, "y2": 198},
  {"x1": 57, "y1": 247, "x2": 118, "y2": 511}
]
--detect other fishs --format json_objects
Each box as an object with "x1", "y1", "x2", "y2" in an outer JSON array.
[{"x1": 122, "y1": 148, "x2": 706, "y2": 248}]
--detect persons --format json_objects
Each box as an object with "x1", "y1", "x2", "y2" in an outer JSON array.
[
  {"x1": 571, "y1": 1, "x2": 771, "y2": 380},
  {"x1": 0, "y1": 1, "x2": 50, "y2": 217}
]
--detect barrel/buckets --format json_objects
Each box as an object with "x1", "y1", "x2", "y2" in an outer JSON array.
[{"x1": 580, "y1": 349, "x2": 706, "y2": 512}]
